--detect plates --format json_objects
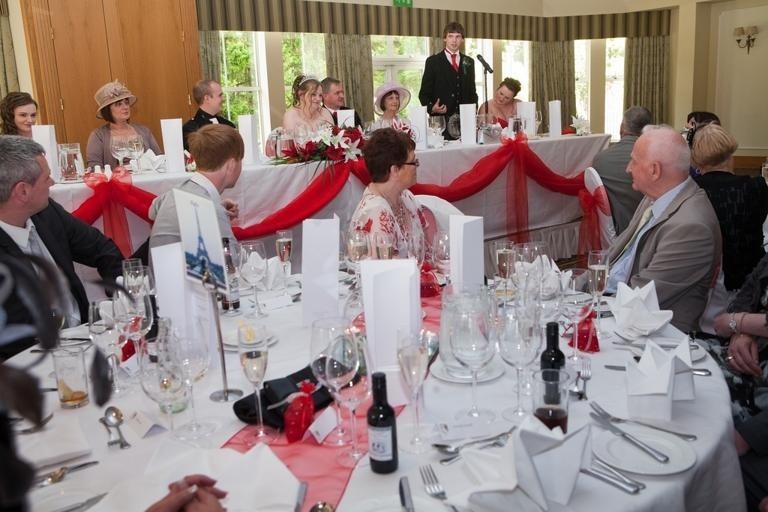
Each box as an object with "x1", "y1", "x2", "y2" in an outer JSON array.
[
  {"x1": 221, "y1": 328, "x2": 278, "y2": 351},
  {"x1": 590, "y1": 424, "x2": 698, "y2": 477},
  {"x1": 429, "y1": 354, "x2": 506, "y2": 382},
  {"x1": 592, "y1": 296, "x2": 616, "y2": 312},
  {"x1": 630, "y1": 337, "x2": 707, "y2": 362}
]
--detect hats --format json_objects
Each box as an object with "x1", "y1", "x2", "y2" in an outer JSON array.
[
  {"x1": 93, "y1": 78, "x2": 137, "y2": 119},
  {"x1": 372, "y1": 81, "x2": 412, "y2": 116}
]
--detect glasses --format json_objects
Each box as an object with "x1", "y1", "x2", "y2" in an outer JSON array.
[{"x1": 401, "y1": 159, "x2": 420, "y2": 167}]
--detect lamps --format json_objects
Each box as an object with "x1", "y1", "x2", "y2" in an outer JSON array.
[{"x1": 732, "y1": 26, "x2": 760, "y2": 55}]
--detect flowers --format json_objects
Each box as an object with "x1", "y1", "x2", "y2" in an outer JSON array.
[{"x1": 266, "y1": 116, "x2": 364, "y2": 184}]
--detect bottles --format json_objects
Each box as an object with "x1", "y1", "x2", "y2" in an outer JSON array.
[
  {"x1": 219, "y1": 237, "x2": 240, "y2": 311},
  {"x1": 368, "y1": 373, "x2": 399, "y2": 473},
  {"x1": 540, "y1": 322, "x2": 564, "y2": 404},
  {"x1": 141, "y1": 295, "x2": 160, "y2": 362}
]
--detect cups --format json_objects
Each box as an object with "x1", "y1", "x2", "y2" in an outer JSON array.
[
  {"x1": 155, "y1": 314, "x2": 217, "y2": 442},
  {"x1": 511, "y1": 240, "x2": 564, "y2": 372},
  {"x1": 560, "y1": 268, "x2": 596, "y2": 360},
  {"x1": 57, "y1": 143, "x2": 81, "y2": 181},
  {"x1": 139, "y1": 336, "x2": 199, "y2": 443},
  {"x1": 122, "y1": 258, "x2": 145, "y2": 301},
  {"x1": 140, "y1": 340, "x2": 185, "y2": 440},
  {"x1": 448, "y1": 300, "x2": 497, "y2": 420},
  {"x1": 431, "y1": 230, "x2": 451, "y2": 294},
  {"x1": 275, "y1": 230, "x2": 293, "y2": 275},
  {"x1": 494, "y1": 240, "x2": 516, "y2": 311},
  {"x1": 587, "y1": 250, "x2": 613, "y2": 340},
  {"x1": 376, "y1": 234, "x2": 393, "y2": 259},
  {"x1": 309, "y1": 315, "x2": 360, "y2": 443},
  {"x1": 346, "y1": 231, "x2": 370, "y2": 280},
  {"x1": 110, "y1": 134, "x2": 130, "y2": 180},
  {"x1": 325, "y1": 337, "x2": 372, "y2": 468},
  {"x1": 123, "y1": 266, "x2": 154, "y2": 304},
  {"x1": 533, "y1": 369, "x2": 570, "y2": 435},
  {"x1": 404, "y1": 231, "x2": 426, "y2": 275},
  {"x1": 397, "y1": 330, "x2": 428, "y2": 446},
  {"x1": 113, "y1": 282, "x2": 156, "y2": 384},
  {"x1": 52, "y1": 346, "x2": 91, "y2": 409},
  {"x1": 237, "y1": 323, "x2": 281, "y2": 446},
  {"x1": 125, "y1": 134, "x2": 148, "y2": 177},
  {"x1": 496, "y1": 296, "x2": 543, "y2": 424},
  {"x1": 239, "y1": 240, "x2": 269, "y2": 319}
]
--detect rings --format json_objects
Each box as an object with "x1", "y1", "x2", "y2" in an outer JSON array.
[{"x1": 725, "y1": 356, "x2": 735, "y2": 361}]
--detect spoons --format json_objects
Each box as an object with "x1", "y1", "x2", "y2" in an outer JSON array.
[
  {"x1": 431, "y1": 423, "x2": 520, "y2": 455},
  {"x1": 104, "y1": 405, "x2": 131, "y2": 449}
]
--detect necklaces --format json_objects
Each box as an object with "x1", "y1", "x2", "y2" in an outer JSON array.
[
  {"x1": 372, "y1": 184, "x2": 407, "y2": 233},
  {"x1": 111, "y1": 122, "x2": 129, "y2": 135}
]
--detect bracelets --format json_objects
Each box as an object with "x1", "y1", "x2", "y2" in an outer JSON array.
[{"x1": 738, "y1": 312, "x2": 748, "y2": 333}]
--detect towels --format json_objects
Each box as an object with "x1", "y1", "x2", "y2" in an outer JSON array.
[
  {"x1": 455, "y1": 411, "x2": 590, "y2": 512},
  {"x1": 612, "y1": 281, "x2": 674, "y2": 339},
  {"x1": 17, "y1": 411, "x2": 91, "y2": 467},
  {"x1": 623, "y1": 336, "x2": 695, "y2": 416}
]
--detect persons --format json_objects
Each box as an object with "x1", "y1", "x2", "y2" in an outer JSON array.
[
  {"x1": 86, "y1": 81, "x2": 162, "y2": 172},
  {"x1": 570, "y1": 127, "x2": 723, "y2": 340},
  {"x1": 0, "y1": 134, "x2": 133, "y2": 365},
  {"x1": 183, "y1": 79, "x2": 237, "y2": 153},
  {"x1": 147, "y1": 124, "x2": 251, "y2": 289},
  {"x1": 418, "y1": 22, "x2": 478, "y2": 140},
  {"x1": 1, "y1": 91, "x2": 38, "y2": 137},
  {"x1": 282, "y1": 73, "x2": 334, "y2": 144},
  {"x1": 366, "y1": 80, "x2": 417, "y2": 139},
  {"x1": 686, "y1": 111, "x2": 721, "y2": 136},
  {"x1": 691, "y1": 122, "x2": 768, "y2": 291},
  {"x1": 734, "y1": 409, "x2": 768, "y2": 512},
  {"x1": 345, "y1": 127, "x2": 430, "y2": 266},
  {"x1": 0, "y1": 362, "x2": 228, "y2": 512},
  {"x1": 684, "y1": 252, "x2": 768, "y2": 423},
  {"x1": 591, "y1": 107, "x2": 656, "y2": 235},
  {"x1": 321, "y1": 77, "x2": 363, "y2": 133},
  {"x1": 479, "y1": 77, "x2": 525, "y2": 126}
]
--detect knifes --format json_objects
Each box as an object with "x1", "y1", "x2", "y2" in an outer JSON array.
[
  {"x1": 611, "y1": 341, "x2": 700, "y2": 349},
  {"x1": 398, "y1": 475, "x2": 415, "y2": 511},
  {"x1": 588, "y1": 411, "x2": 670, "y2": 463},
  {"x1": 293, "y1": 482, "x2": 307, "y2": 512},
  {"x1": 604, "y1": 365, "x2": 712, "y2": 376}
]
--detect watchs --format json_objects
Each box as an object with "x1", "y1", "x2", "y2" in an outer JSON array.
[{"x1": 727, "y1": 312, "x2": 737, "y2": 335}]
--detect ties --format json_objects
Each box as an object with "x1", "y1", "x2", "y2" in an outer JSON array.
[
  {"x1": 27, "y1": 230, "x2": 49, "y2": 281},
  {"x1": 609, "y1": 204, "x2": 651, "y2": 268},
  {"x1": 444, "y1": 48, "x2": 460, "y2": 73}
]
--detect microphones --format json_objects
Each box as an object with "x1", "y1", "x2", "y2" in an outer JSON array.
[{"x1": 476, "y1": 54, "x2": 493, "y2": 73}]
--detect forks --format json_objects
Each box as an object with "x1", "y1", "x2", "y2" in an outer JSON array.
[
  {"x1": 579, "y1": 357, "x2": 592, "y2": 401},
  {"x1": 13, "y1": 411, "x2": 54, "y2": 435},
  {"x1": 438, "y1": 432, "x2": 512, "y2": 466},
  {"x1": 419, "y1": 464, "x2": 459, "y2": 512},
  {"x1": 99, "y1": 417, "x2": 121, "y2": 448},
  {"x1": 588, "y1": 400, "x2": 698, "y2": 442}
]
